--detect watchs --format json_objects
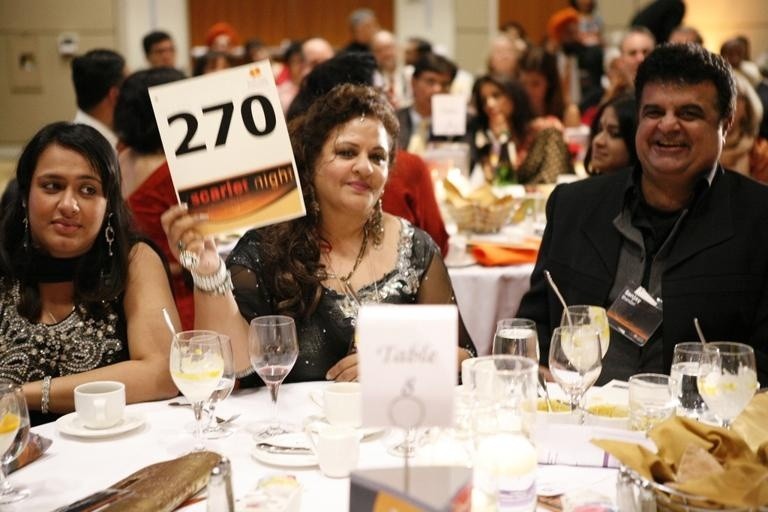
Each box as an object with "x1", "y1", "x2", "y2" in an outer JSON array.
[{"x1": 498, "y1": 131, "x2": 514, "y2": 145}]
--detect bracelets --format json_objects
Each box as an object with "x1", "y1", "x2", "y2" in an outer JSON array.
[
  {"x1": 41, "y1": 373, "x2": 51, "y2": 414},
  {"x1": 189, "y1": 257, "x2": 234, "y2": 295}
]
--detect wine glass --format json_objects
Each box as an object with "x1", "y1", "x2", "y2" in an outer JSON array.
[
  {"x1": 494, "y1": 320, "x2": 539, "y2": 395},
  {"x1": 462, "y1": 357, "x2": 536, "y2": 443},
  {"x1": 0, "y1": 378, "x2": 30, "y2": 506},
  {"x1": 169, "y1": 330, "x2": 224, "y2": 453},
  {"x1": 549, "y1": 326, "x2": 603, "y2": 412},
  {"x1": 627, "y1": 374, "x2": 674, "y2": 430},
  {"x1": 561, "y1": 305, "x2": 610, "y2": 395},
  {"x1": 670, "y1": 342, "x2": 720, "y2": 417},
  {"x1": 247, "y1": 316, "x2": 299, "y2": 406},
  {"x1": 703, "y1": 341, "x2": 756, "y2": 432},
  {"x1": 189, "y1": 335, "x2": 235, "y2": 438}
]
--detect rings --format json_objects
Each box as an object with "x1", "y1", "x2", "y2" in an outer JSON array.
[{"x1": 177, "y1": 240, "x2": 185, "y2": 251}]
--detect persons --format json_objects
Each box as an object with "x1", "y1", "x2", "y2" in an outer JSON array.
[
  {"x1": 720, "y1": 39, "x2": 757, "y2": 90},
  {"x1": 532, "y1": 10, "x2": 604, "y2": 111},
  {"x1": 720, "y1": 68, "x2": 768, "y2": 184},
  {"x1": 111, "y1": 68, "x2": 194, "y2": 329},
  {"x1": 583, "y1": 95, "x2": 640, "y2": 177},
  {"x1": 507, "y1": 43, "x2": 768, "y2": 408},
  {"x1": 468, "y1": 73, "x2": 575, "y2": 186},
  {"x1": 608, "y1": 28, "x2": 659, "y2": 112},
  {"x1": 159, "y1": 83, "x2": 479, "y2": 384},
  {"x1": 405, "y1": 37, "x2": 433, "y2": 68},
  {"x1": 195, "y1": 22, "x2": 336, "y2": 116},
  {"x1": 393, "y1": 52, "x2": 472, "y2": 151},
  {"x1": 486, "y1": 22, "x2": 525, "y2": 74},
  {"x1": 666, "y1": 28, "x2": 764, "y2": 173},
  {"x1": 367, "y1": 30, "x2": 417, "y2": 110},
  {"x1": 0, "y1": 120, "x2": 183, "y2": 429},
  {"x1": 284, "y1": 59, "x2": 450, "y2": 259},
  {"x1": 336, "y1": 7, "x2": 381, "y2": 55},
  {"x1": 142, "y1": 32, "x2": 177, "y2": 68},
  {"x1": 569, "y1": 0, "x2": 605, "y2": 47},
  {"x1": 753, "y1": 58, "x2": 768, "y2": 142},
  {"x1": 516, "y1": 46, "x2": 590, "y2": 158},
  {"x1": 67, "y1": 49, "x2": 125, "y2": 154}
]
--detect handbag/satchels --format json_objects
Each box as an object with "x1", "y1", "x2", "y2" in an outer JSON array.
[{"x1": 53, "y1": 452, "x2": 221, "y2": 512}]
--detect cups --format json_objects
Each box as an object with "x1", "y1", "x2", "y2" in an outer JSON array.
[
  {"x1": 309, "y1": 382, "x2": 361, "y2": 424},
  {"x1": 308, "y1": 425, "x2": 361, "y2": 476},
  {"x1": 73, "y1": 379, "x2": 125, "y2": 428}
]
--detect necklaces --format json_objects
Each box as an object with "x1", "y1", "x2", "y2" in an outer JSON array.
[{"x1": 315, "y1": 220, "x2": 370, "y2": 281}]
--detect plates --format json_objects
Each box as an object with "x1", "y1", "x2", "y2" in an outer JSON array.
[
  {"x1": 54, "y1": 409, "x2": 139, "y2": 438},
  {"x1": 255, "y1": 428, "x2": 320, "y2": 468}
]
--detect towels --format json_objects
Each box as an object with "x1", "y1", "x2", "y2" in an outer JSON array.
[
  {"x1": 536, "y1": 439, "x2": 621, "y2": 470},
  {"x1": 588, "y1": 413, "x2": 767, "y2": 509},
  {"x1": 442, "y1": 176, "x2": 514, "y2": 215}
]
